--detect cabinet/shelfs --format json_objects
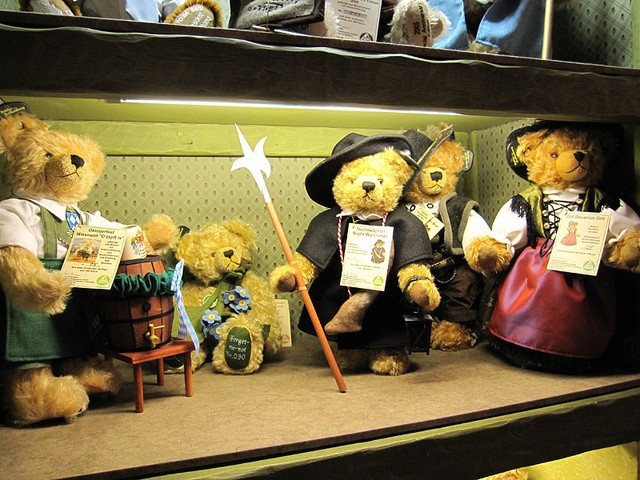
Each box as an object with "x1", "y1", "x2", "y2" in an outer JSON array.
[{"x1": 1, "y1": 10, "x2": 640, "y2": 480}]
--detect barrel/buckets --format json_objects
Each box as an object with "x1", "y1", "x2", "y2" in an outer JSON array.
[{"x1": 100, "y1": 255, "x2": 174, "y2": 351}]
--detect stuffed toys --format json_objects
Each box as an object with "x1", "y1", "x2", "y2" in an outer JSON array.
[
  {"x1": 165, "y1": 216, "x2": 282, "y2": 377},
  {"x1": 266, "y1": 129, "x2": 442, "y2": 377},
  {"x1": 561, "y1": 221, "x2": 582, "y2": 247},
  {"x1": 395, "y1": 122, "x2": 507, "y2": 353},
  {"x1": 76, "y1": 240, "x2": 93, "y2": 261},
  {"x1": 370, "y1": 239, "x2": 387, "y2": 265},
  {"x1": 0, "y1": 109, "x2": 181, "y2": 428},
  {"x1": 486, "y1": 120, "x2": 640, "y2": 378}
]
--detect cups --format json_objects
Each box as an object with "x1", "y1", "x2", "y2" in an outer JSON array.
[{"x1": 119, "y1": 224, "x2": 146, "y2": 261}]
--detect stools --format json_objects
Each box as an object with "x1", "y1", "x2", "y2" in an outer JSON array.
[{"x1": 100, "y1": 335, "x2": 195, "y2": 413}]
